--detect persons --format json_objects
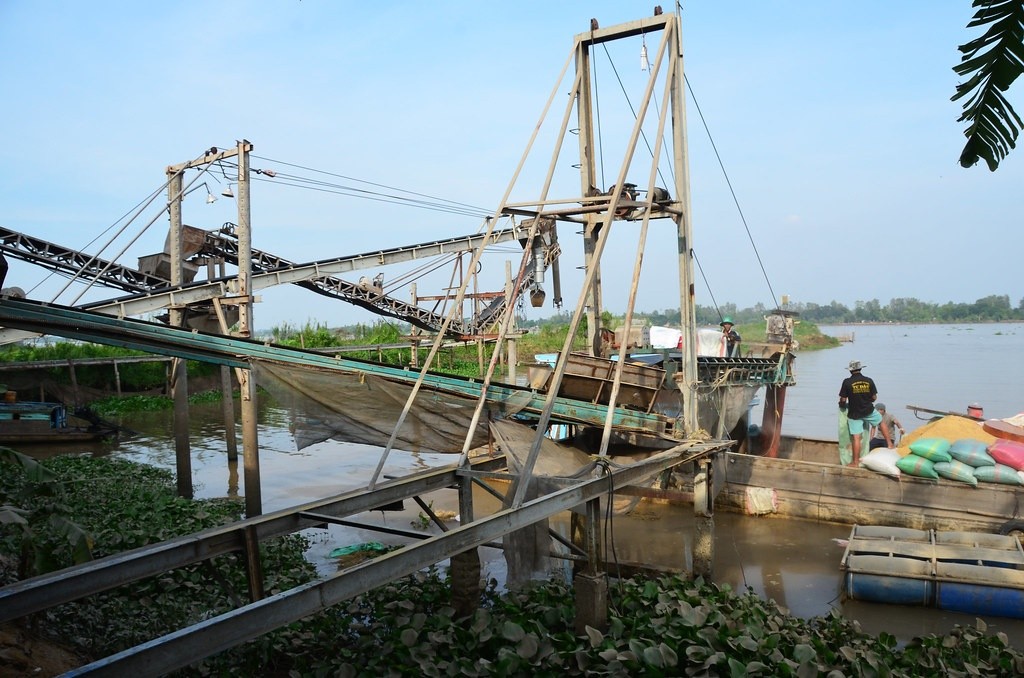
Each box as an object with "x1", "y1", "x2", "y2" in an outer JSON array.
[
  {"x1": 720, "y1": 317, "x2": 741, "y2": 357},
  {"x1": 839, "y1": 361, "x2": 905, "y2": 468}
]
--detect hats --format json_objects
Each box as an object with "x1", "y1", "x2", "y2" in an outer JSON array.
[
  {"x1": 720, "y1": 315, "x2": 735, "y2": 326},
  {"x1": 873, "y1": 403, "x2": 885, "y2": 411},
  {"x1": 845, "y1": 361, "x2": 867, "y2": 371}
]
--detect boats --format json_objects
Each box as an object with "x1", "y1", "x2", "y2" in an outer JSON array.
[
  {"x1": 1, "y1": 403, "x2": 126, "y2": 441},
  {"x1": 546, "y1": 423, "x2": 1024, "y2": 532},
  {"x1": 837, "y1": 524, "x2": 1024, "y2": 621}
]
